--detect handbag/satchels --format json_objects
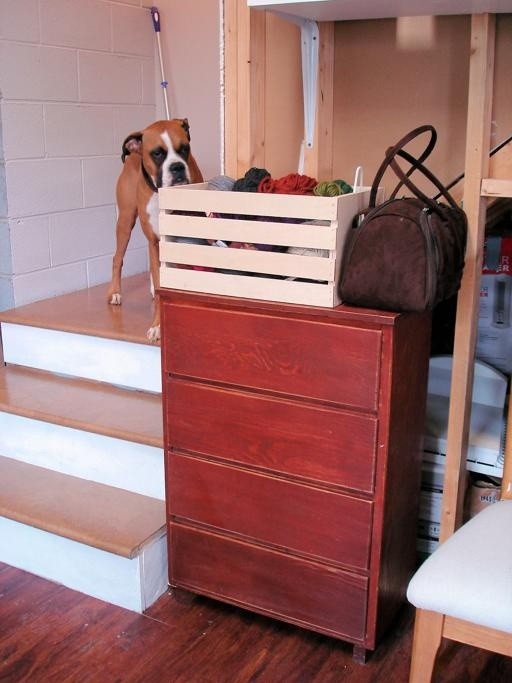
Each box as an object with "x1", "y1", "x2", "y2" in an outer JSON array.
[{"x1": 336, "y1": 124, "x2": 468, "y2": 316}]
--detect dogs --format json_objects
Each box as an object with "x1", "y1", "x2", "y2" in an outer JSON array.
[{"x1": 106, "y1": 118, "x2": 205, "y2": 346}]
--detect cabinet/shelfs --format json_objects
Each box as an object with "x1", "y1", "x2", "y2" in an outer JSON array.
[{"x1": 154, "y1": 286, "x2": 430, "y2": 668}]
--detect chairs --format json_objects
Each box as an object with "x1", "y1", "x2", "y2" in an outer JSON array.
[{"x1": 404, "y1": 375, "x2": 509, "y2": 683}]
